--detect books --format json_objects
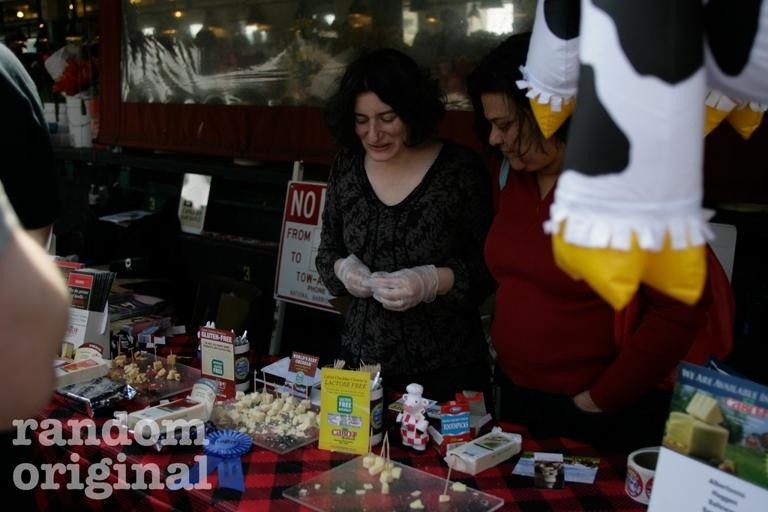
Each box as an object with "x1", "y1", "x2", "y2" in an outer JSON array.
[{"x1": 48, "y1": 262, "x2": 199, "y2": 390}]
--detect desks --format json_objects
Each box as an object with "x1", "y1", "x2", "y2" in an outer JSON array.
[{"x1": 33, "y1": 328, "x2": 645, "y2": 511}]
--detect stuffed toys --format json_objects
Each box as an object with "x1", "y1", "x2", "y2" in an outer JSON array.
[
  {"x1": 511, "y1": 0, "x2": 768, "y2": 311},
  {"x1": 395, "y1": 381, "x2": 430, "y2": 451}
]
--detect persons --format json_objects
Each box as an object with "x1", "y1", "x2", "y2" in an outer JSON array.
[
  {"x1": 314, "y1": 47, "x2": 496, "y2": 423},
  {"x1": 1, "y1": 180, "x2": 72, "y2": 438},
  {"x1": 0, "y1": 39, "x2": 56, "y2": 252},
  {"x1": 462, "y1": 31, "x2": 715, "y2": 464}
]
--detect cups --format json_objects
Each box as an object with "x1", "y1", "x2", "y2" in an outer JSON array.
[{"x1": 234, "y1": 339, "x2": 250, "y2": 393}]
